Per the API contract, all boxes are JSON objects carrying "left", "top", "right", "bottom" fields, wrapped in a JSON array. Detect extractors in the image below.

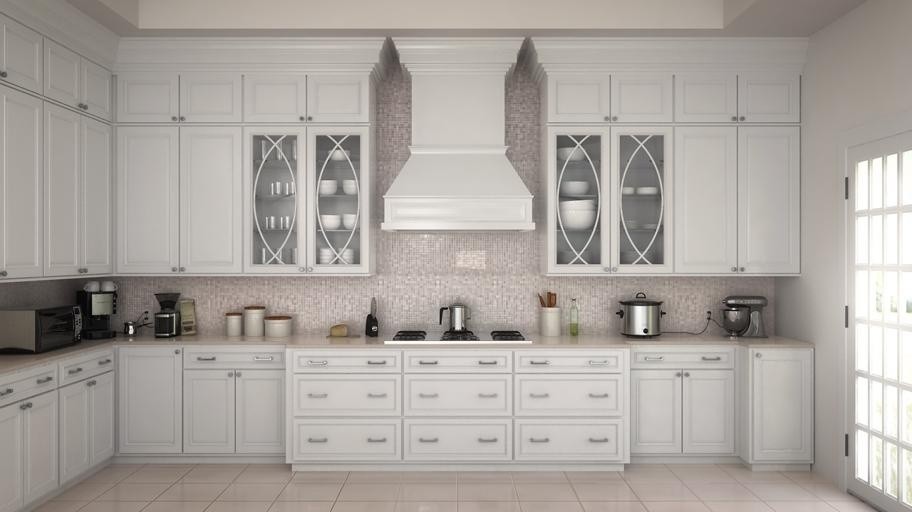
[{"left": 381, "top": 38, "right": 537, "bottom": 232}]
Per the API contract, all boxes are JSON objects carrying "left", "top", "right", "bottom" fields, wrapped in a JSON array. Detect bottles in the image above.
[
  {"left": 569, "top": 297, "right": 580, "bottom": 337},
  {"left": 226, "top": 312, "right": 242, "bottom": 337},
  {"left": 244, "top": 306, "right": 265, "bottom": 336}
]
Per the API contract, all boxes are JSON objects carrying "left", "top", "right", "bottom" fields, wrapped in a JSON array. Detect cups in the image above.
[
  {"left": 84, "top": 279, "right": 99, "bottom": 291},
  {"left": 101, "top": 280, "right": 117, "bottom": 292},
  {"left": 540, "top": 307, "right": 562, "bottom": 337},
  {"left": 343, "top": 213, "right": 359, "bottom": 230}
]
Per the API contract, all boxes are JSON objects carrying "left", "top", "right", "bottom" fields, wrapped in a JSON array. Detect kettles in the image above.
[{"left": 124, "top": 320, "right": 137, "bottom": 337}]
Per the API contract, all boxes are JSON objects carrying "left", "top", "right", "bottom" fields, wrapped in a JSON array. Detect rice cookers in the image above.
[{"left": 616, "top": 291, "right": 666, "bottom": 339}]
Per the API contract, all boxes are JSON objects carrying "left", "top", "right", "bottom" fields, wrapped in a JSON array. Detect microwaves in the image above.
[{"left": 0, "top": 304, "right": 83, "bottom": 355}]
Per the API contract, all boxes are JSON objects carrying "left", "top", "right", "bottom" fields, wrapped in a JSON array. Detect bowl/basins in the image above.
[
  {"left": 319, "top": 178, "right": 337, "bottom": 196},
  {"left": 320, "top": 248, "right": 335, "bottom": 264},
  {"left": 321, "top": 213, "right": 340, "bottom": 229},
  {"left": 623, "top": 220, "right": 638, "bottom": 230},
  {"left": 559, "top": 146, "right": 585, "bottom": 162},
  {"left": 339, "top": 248, "right": 354, "bottom": 265},
  {"left": 620, "top": 187, "right": 658, "bottom": 196},
  {"left": 560, "top": 251, "right": 592, "bottom": 265},
  {"left": 343, "top": 178, "right": 358, "bottom": 195},
  {"left": 328, "top": 148, "right": 350, "bottom": 161},
  {"left": 559, "top": 199, "right": 594, "bottom": 230},
  {"left": 560, "top": 180, "right": 590, "bottom": 197},
  {"left": 620, "top": 249, "right": 657, "bottom": 264}
]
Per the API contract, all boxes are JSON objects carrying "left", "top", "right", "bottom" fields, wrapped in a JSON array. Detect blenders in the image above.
[{"left": 719, "top": 295, "right": 769, "bottom": 341}]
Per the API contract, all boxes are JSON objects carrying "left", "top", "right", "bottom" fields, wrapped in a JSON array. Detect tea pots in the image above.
[{"left": 439, "top": 302, "right": 473, "bottom": 332}]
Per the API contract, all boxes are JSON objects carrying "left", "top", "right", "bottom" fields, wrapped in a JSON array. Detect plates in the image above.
[{"left": 644, "top": 223, "right": 660, "bottom": 229}]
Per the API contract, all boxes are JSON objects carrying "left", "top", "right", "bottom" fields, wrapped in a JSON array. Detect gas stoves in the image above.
[{"left": 392, "top": 330, "right": 524, "bottom": 340}]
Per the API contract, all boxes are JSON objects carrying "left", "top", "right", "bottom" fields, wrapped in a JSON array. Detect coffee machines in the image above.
[
  {"left": 76, "top": 291, "right": 117, "bottom": 339},
  {"left": 151, "top": 291, "right": 181, "bottom": 337}
]
